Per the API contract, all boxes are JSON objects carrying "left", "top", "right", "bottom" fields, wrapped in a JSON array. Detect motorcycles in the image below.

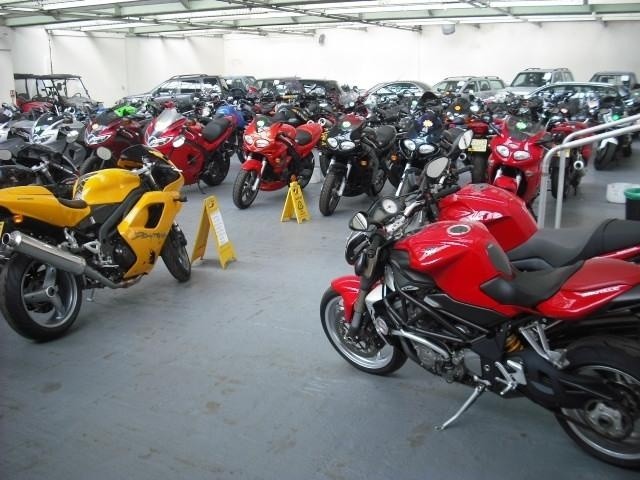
[
  {"left": 384, "top": 122, "right": 640, "bottom": 270},
  {"left": 0, "top": 131, "right": 194, "bottom": 344},
  {"left": 312, "top": 179, "right": 640, "bottom": 472}
]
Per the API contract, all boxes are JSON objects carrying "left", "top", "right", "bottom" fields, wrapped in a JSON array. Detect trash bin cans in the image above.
[{"left": 624, "top": 187, "right": 640, "bottom": 221}]
